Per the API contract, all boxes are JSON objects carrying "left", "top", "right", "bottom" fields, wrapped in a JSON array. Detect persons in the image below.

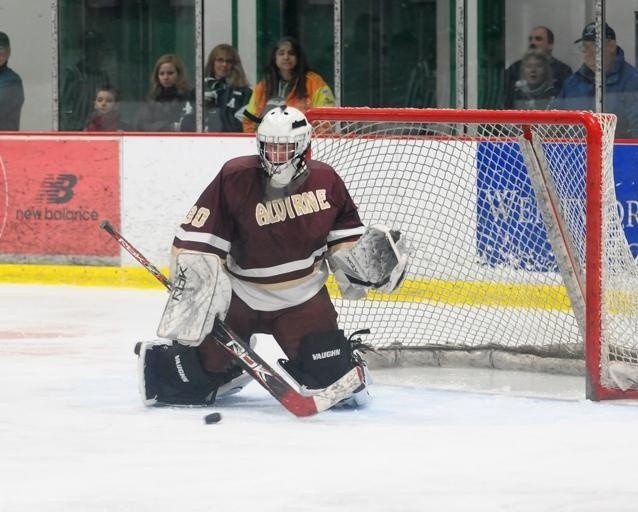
[
  {"left": 192, "top": 44, "right": 251, "bottom": 135},
  {"left": 507, "top": 50, "right": 557, "bottom": 111},
  {"left": 135, "top": 108, "right": 408, "bottom": 410},
  {"left": 0, "top": 29, "right": 25, "bottom": 131},
  {"left": 78, "top": 85, "right": 123, "bottom": 130},
  {"left": 557, "top": 22, "right": 638, "bottom": 98},
  {"left": 235, "top": 38, "right": 337, "bottom": 134},
  {"left": 150, "top": 55, "right": 197, "bottom": 102},
  {"left": 505, "top": 27, "right": 572, "bottom": 95}
]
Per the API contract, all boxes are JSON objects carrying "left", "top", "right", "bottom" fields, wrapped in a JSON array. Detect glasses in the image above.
[{"left": 215, "top": 56, "right": 232, "bottom": 65}]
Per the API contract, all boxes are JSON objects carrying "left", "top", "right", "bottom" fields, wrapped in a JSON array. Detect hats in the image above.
[{"left": 574, "top": 22, "right": 615, "bottom": 44}]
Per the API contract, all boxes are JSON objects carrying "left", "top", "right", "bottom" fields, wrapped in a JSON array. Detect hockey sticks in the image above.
[{"left": 100, "top": 220, "right": 364, "bottom": 418}]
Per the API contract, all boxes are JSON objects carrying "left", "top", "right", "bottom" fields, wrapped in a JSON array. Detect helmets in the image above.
[{"left": 257, "top": 108, "right": 311, "bottom": 182}]
